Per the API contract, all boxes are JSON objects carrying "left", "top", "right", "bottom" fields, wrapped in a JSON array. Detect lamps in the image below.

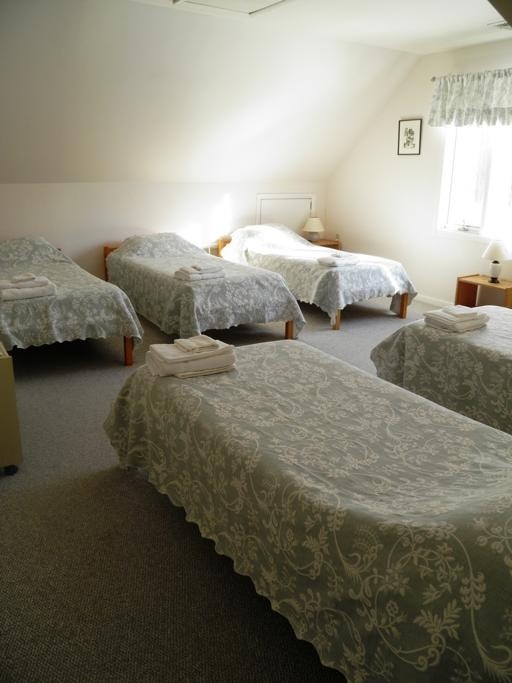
[
  {"left": 302, "top": 217, "right": 325, "bottom": 241},
  {"left": 480, "top": 238, "right": 509, "bottom": 283}
]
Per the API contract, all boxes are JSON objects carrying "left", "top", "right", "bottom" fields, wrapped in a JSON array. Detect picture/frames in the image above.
[{"left": 398, "top": 116, "right": 421, "bottom": 155}]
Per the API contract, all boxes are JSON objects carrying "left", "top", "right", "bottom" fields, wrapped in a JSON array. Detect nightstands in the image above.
[{"left": 455, "top": 273, "right": 510, "bottom": 308}]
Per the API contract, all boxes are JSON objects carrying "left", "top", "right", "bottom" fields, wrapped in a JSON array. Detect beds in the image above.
[
  {"left": 1, "top": 233, "right": 142, "bottom": 368},
  {"left": 368, "top": 302, "right": 512, "bottom": 434},
  {"left": 217, "top": 223, "right": 418, "bottom": 331},
  {"left": 102, "top": 232, "right": 307, "bottom": 342},
  {"left": 102, "top": 339, "right": 509, "bottom": 682}
]
